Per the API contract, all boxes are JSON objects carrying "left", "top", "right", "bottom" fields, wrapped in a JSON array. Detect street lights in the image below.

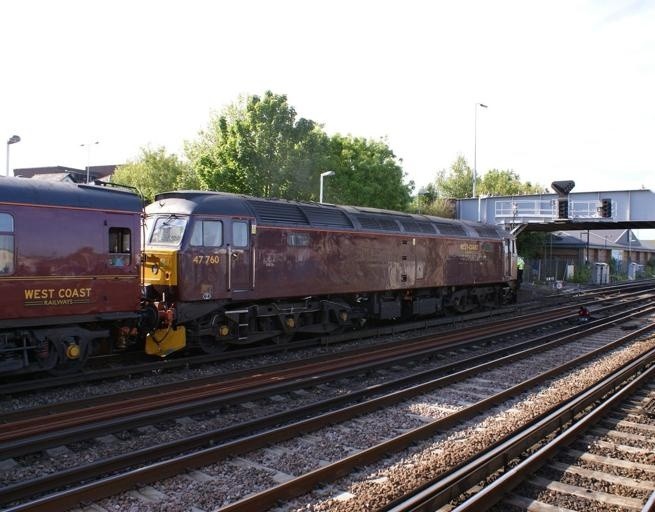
[
  {"left": 81, "top": 139, "right": 100, "bottom": 183},
  {"left": 6, "top": 135, "right": 22, "bottom": 177},
  {"left": 317, "top": 171, "right": 336, "bottom": 204},
  {"left": 473, "top": 100, "right": 490, "bottom": 222}
]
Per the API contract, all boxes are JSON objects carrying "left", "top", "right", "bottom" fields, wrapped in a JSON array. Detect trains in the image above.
[{"left": 0, "top": 177, "right": 519, "bottom": 375}]
